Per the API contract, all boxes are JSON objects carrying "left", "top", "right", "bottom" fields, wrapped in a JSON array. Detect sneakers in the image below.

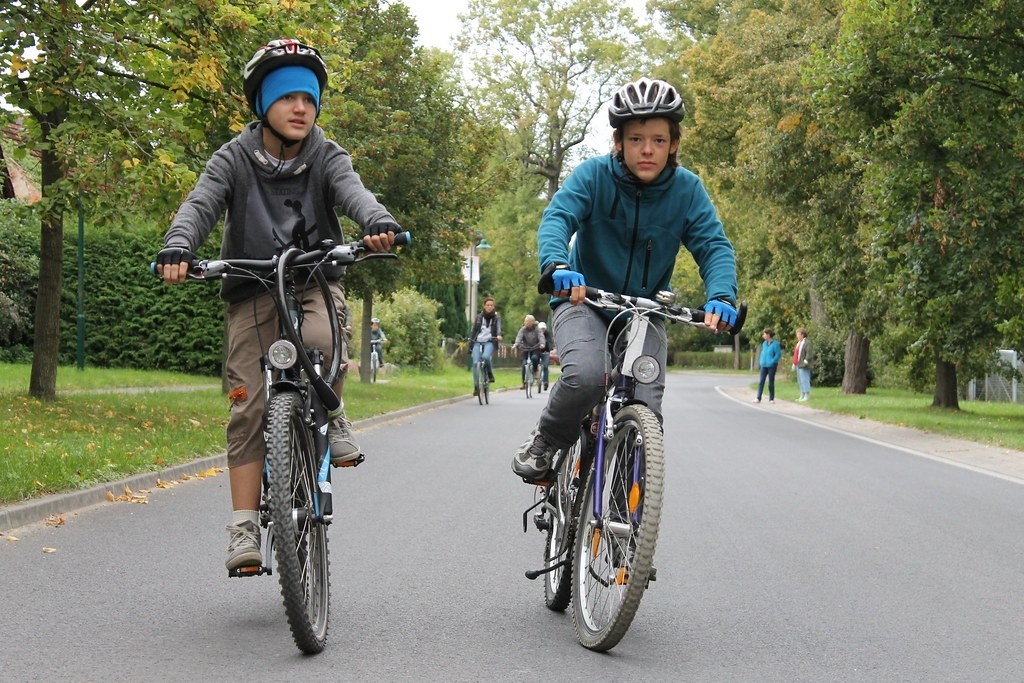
[
  {"left": 511, "top": 417, "right": 559, "bottom": 480},
  {"left": 606, "top": 534, "right": 658, "bottom": 578},
  {"left": 327, "top": 408, "right": 361, "bottom": 462},
  {"left": 225, "top": 519, "right": 263, "bottom": 571}
]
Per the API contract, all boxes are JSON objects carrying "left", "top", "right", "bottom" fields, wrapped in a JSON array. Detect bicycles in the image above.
[
  {"left": 522, "top": 261, "right": 747, "bottom": 651},
  {"left": 517, "top": 342, "right": 554, "bottom": 398},
  {"left": 151, "top": 231, "right": 411, "bottom": 655},
  {"left": 463, "top": 338, "right": 498, "bottom": 405},
  {"left": 371, "top": 339, "right": 386, "bottom": 382}
]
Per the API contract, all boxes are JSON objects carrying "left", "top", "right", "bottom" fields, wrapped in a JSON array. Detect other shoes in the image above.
[
  {"left": 535, "top": 371, "right": 540, "bottom": 379},
  {"left": 490, "top": 377, "right": 494, "bottom": 382},
  {"left": 544, "top": 383, "right": 548, "bottom": 390},
  {"left": 520, "top": 383, "right": 526, "bottom": 390},
  {"left": 474, "top": 389, "right": 480, "bottom": 396},
  {"left": 796, "top": 394, "right": 810, "bottom": 402}
]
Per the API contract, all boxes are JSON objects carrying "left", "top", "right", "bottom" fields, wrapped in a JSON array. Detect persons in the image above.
[
  {"left": 512, "top": 314, "right": 545, "bottom": 390},
  {"left": 469, "top": 298, "right": 502, "bottom": 396},
  {"left": 534, "top": 322, "right": 553, "bottom": 390},
  {"left": 511, "top": 78, "right": 739, "bottom": 581},
  {"left": 368, "top": 318, "right": 389, "bottom": 370},
  {"left": 753, "top": 330, "right": 781, "bottom": 404},
  {"left": 156, "top": 38, "right": 402, "bottom": 571},
  {"left": 792, "top": 328, "right": 812, "bottom": 402}
]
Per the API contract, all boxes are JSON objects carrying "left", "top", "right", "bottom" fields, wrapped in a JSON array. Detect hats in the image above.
[
  {"left": 255, "top": 66, "right": 320, "bottom": 119},
  {"left": 538, "top": 322, "right": 546, "bottom": 329}
]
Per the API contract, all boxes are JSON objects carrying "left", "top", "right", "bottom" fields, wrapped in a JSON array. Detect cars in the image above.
[{"left": 549, "top": 349, "right": 559, "bottom": 365}]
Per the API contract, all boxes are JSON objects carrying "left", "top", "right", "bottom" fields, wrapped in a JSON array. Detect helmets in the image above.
[
  {"left": 371, "top": 318, "right": 380, "bottom": 324},
  {"left": 609, "top": 77, "right": 685, "bottom": 128},
  {"left": 243, "top": 39, "right": 327, "bottom": 115}
]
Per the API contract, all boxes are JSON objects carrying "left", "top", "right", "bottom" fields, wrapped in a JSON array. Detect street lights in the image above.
[{"left": 467, "top": 231, "right": 492, "bottom": 371}]
